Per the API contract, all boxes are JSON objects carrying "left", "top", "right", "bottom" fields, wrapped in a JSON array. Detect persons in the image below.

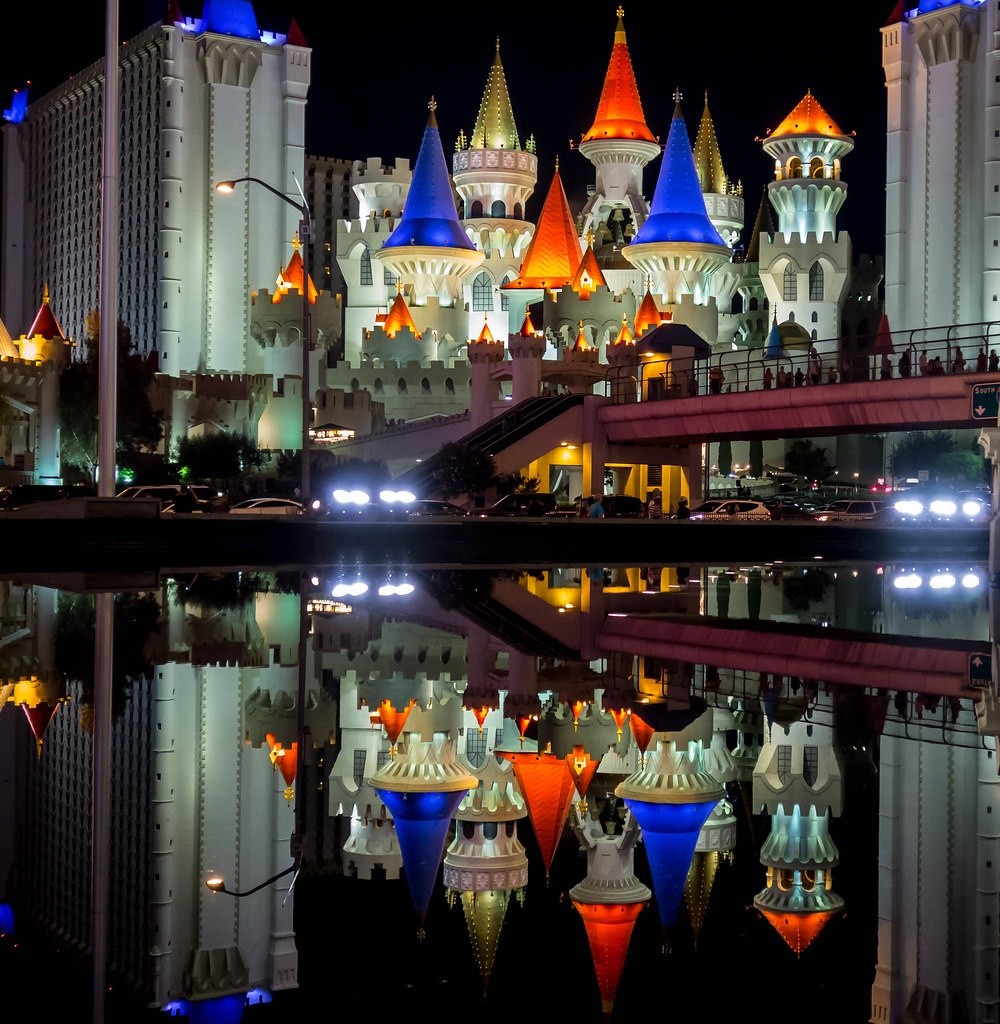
[
  {"left": 543, "top": 388, "right": 573, "bottom": 397},
  {"left": 878, "top": 347, "right": 1000, "bottom": 378},
  {"left": 649, "top": 493, "right": 664, "bottom": 519},
  {"left": 684, "top": 360, "right": 839, "bottom": 395},
  {"left": 677, "top": 496, "right": 690, "bottom": 520},
  {"left": 382, "top": 613, "right": 466, "bottom": 642},
  {"left": 536, "top": 656, "right": 569, "bottom": 668},
  {"left": 171, "top": 484, "right": 302, "bottom": 513},
  {"left": 384, "top": 408, "right": 470, "bottom": 431},
  {"left": 657, "top": 658, "right": 977, "bottom": 725},
  {"left": 166, "top": 573, "right": 302, "bottom": 599},
  {"left": 588, "top": 492, "right": 606, "bottom": 521}
]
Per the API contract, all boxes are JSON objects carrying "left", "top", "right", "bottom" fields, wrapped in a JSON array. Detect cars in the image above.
[
  {"left": 115, "top": 484, "right": 211, "bottom": 504},
  {"left": 566, "top": 494, "right": 643, "bottom": 516},
  {"left": 815, "top": 500, "right": 885, "bottom": 522},
  {"left": 161, "top": 499, "right": 232, "bottom": 513},
  {"left": 384, "top": 500, "right": 468, "bottom": 517},
  {"left": 467, "top": 493, "right": 559, "bottom": 518},
  {"left": 0, "top": 484, "right": 95, "bottom": 509},
  {"left": 958, "top": 487, "right": 991, "bottom": 495},
  {"left": 803, "top": 503, "right": 837, "bottom": 516},
  {"left": 671, "top": 499, "right": 771, "bottom": 520},
  {"left": 229, "top": 498, "right": 302, "bottom": 514}
]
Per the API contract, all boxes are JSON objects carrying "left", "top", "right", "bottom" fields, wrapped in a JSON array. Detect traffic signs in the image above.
[
  {"left": 969, "top": 652, "right": 993, "bottom": 691},
  {"left": 972, "top": 383, "right": 1000, "bottom": 419}
]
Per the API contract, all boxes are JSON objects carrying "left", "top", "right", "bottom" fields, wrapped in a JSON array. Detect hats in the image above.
[
  {"left": 594, "top": 494, "right": 603, "bottom": 500},
  {"left": 679, "top": 496, "right": 687, "bottom": 502},
  {"left": 653, "top": 488, "right": 662, "bottom": 497}
]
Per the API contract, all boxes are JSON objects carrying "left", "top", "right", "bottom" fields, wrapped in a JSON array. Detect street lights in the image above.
[
  {"left": 854, "top": 473, "right": 859, "bottom": 493},
  {"left": 215, "top": 176, "right": 311, "bottom": 508},
  {"left": 835, "top": 471, "right": 838, "bottom": 495},
  {"left": 205, "top": 572, "right": 309, "bottom": 897}
]
